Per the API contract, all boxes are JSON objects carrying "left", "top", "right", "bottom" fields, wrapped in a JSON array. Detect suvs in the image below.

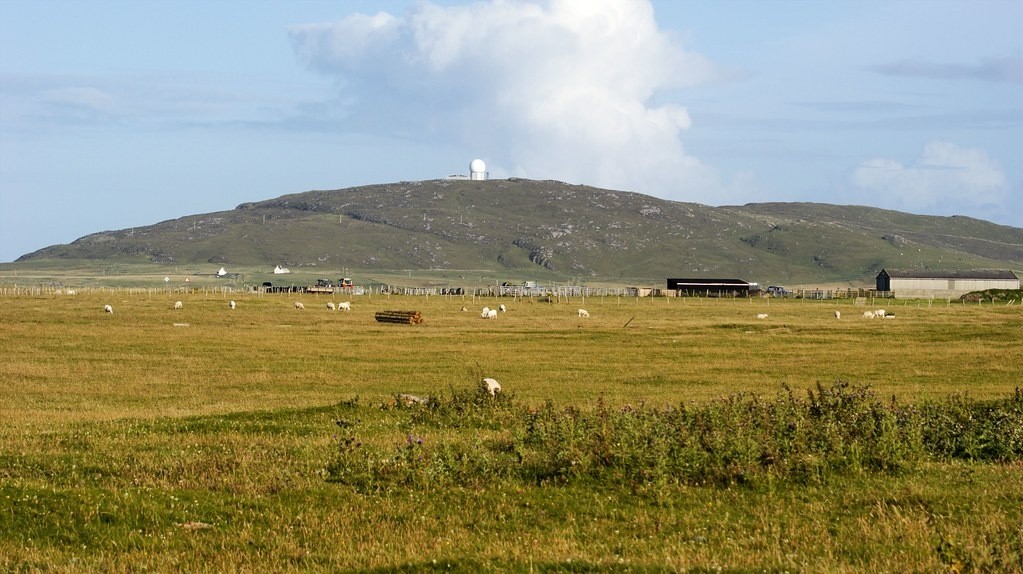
[{"left": 767, "top": 285, "right": 793, "bottom": 298}]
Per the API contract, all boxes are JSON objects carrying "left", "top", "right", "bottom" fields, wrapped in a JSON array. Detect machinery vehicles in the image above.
[
  {"left": 308, "top": 278, "right": 333, "bottom": 293},
  {"left": 339, "top": 277, "right": 352, "bottom": 288}
]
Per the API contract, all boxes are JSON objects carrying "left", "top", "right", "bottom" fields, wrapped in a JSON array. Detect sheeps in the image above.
[
  {"left": 756, "top": 314, "right": 768, "bottom": 319},
  {"left": 174, "top": 300, "right": 182, "bottom": 309},
  {"left": 834, "top": 311, "right": 840, "bottom": 319},
  {"left": 105, "top": 305, "right": 113, "bottom": 314},
  {"left": 499, "top": 304, "right": 506, "bottom": 313},
  {"left": 488, "top": 310, "right": 497, "bottom": 319},
  {"left": 326, "top": 302, "right": 335, "bottom": 310},
  {"left": 861, "top": 311, "right": 873, "bottom": 319},
  {"left": 229, "top": 301, "right": 236, "bottom": 309},
  {"left": 578, "top": 309, "right": 589, "bottom": 318},
  {"left": 338, "top": 303, "right": 350, "bottom": 311},
  {"left": 295, "top": 302, "right": 304, "bottom": 310},
  {"left": 480, "top": 307, "right": 489, "bottom": 317},
  {"left": 872, "top": 310, "right": 885, "bottom": 318}
]
